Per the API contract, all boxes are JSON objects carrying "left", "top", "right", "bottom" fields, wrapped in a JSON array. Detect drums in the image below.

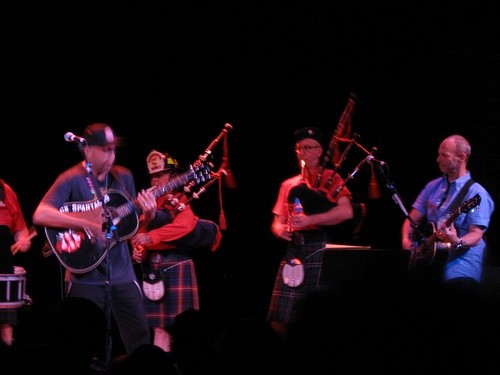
[{"left": 1, "top": 262, "right": 28, "bottom": 310}]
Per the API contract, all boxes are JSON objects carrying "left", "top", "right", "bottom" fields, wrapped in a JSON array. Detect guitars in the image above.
[
  {"left": 409, "top": 193, "right": 482, "bottom": 274},
  {"left": 44, "top": 163, "right": 213, "bottom": 276}
]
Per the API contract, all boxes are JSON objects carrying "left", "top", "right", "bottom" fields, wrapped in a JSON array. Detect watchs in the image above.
[{"left": 456, "top": 239, "right": 464, "bottom": 249}]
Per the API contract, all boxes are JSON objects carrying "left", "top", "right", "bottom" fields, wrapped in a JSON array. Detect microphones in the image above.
[
  {"left": 63, "top": 132, "right": 86, "bottom": 143},
  {"left": 365, "top": 156, "right": 385, "bottom": 166}
]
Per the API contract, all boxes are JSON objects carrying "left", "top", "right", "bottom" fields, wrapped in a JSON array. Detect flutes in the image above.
[
  {"left": 145, "top": 122, "right": 236, "bottom": 232},
  {"left": 285, "top": 93, "right": 385, "bottom": 246}
]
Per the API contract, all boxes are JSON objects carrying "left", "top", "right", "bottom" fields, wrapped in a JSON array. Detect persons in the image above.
[
  {"left": 33, "top": 124, "right": 158, "bottom": 371},
  {"left": 130, "top": 149, "right": 199, "bottom": 352},
  {"left": 402, "top": 135, "right": 494, "bottom": 283},
  {"left": 266, "top": 129, "right": 353, "bottom": 333},
  {"left": 0, "top": 180, "right": 30, "bottom": 345}
]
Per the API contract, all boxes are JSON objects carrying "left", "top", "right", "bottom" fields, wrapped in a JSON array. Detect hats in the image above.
[
  {"left": 82, "top": 124, "right": 124, "bottom": 146},
  {"left": 294, "top": 126, "right": 326, "bottom": 150},
  {"left": 145, "top": 149, "right": 179, "bottom": 176}
]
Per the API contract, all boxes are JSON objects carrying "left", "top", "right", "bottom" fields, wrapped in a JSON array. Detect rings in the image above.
[{"left": 144, "top": 202, "right": 149, "bottom": 207}]
